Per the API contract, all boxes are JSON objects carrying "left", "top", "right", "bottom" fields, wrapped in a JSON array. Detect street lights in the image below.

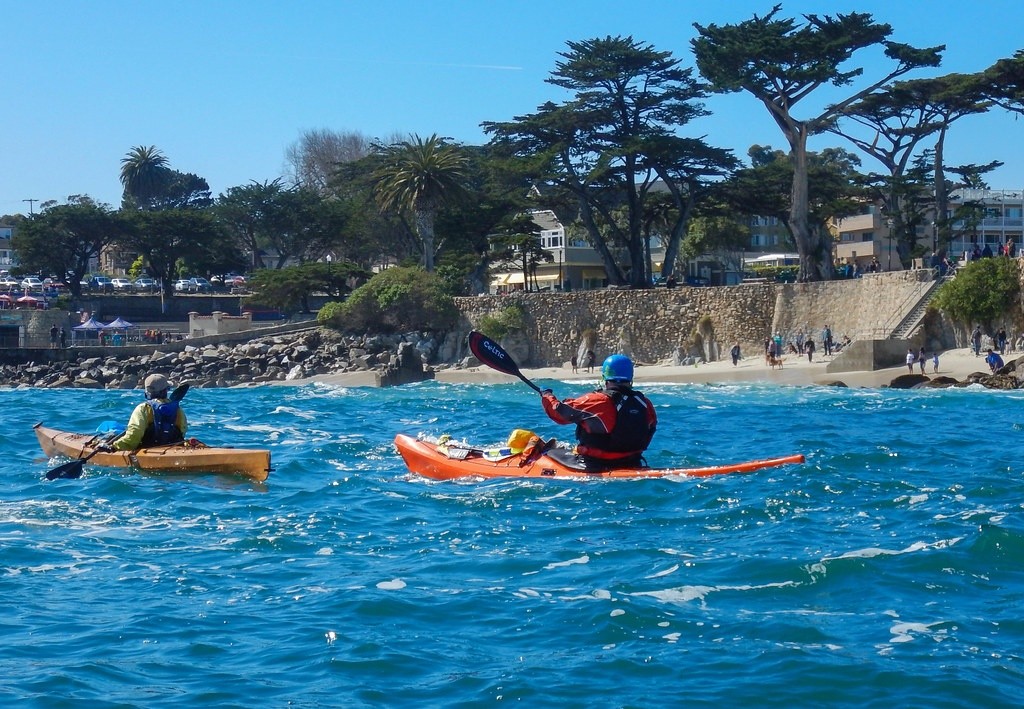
[
  {"left": 931, "top": 222, "right": 936, "bottom": 253},
  {"left": 326, "top": 254, "right": 332, "bottom": 281},
  {"left": 247, "top": 251, "right": 254, "bottom": 264},
  {"left": 559, "top": 247, "right": 562, "bottom": 290},
  {"left": 888, "top": 219, "right": 893, "bottom": 272},
  {"left": 103, "top": 269, "right": 108, "bottom": 295}
]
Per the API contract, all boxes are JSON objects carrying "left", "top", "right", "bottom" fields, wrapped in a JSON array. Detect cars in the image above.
[{"left": 0, "top": 269, "right": 251, "bottom": 293}]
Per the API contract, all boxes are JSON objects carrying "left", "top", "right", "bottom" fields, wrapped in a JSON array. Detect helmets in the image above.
[{"left": 601, "top": 354, "right": 634, "bottom": 381}]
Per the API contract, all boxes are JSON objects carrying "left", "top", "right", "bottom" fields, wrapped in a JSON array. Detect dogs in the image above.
[{"left": 767, "top": 353, "right": 787, "bottom": 370}]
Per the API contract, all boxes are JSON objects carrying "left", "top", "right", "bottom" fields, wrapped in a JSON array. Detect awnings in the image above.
[
  {"left": 490, "top": 269, "right": 559, "bottom": 286},
  {"left": 583, "top": 269, "right": 606, "bottom": 278}
]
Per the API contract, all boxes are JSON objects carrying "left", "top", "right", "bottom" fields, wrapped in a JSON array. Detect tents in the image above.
[{"left": 72, "top": 314, "right": 133, "bottom": 339}]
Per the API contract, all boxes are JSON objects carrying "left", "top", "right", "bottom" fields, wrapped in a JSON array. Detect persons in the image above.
[
  {"left": 787, "top": 342, "right": 797, "bottom": 354},
  {"left": 730, "top": 342, "right": 740, "bottom": 365},
  {"left": 571, "top": 355, "right": 578, "bottom": 374},
  {"left": 50, "top": 324, "right": 66, "bottom": 349},
  {"left": 822, "top": 324, "right": 850, "bottom": 355},
  {"left": 845, "top": 256, "right": 881, "bottom": 279},
  {"left": 667, "top": 275, "right": 677, "bottom": 288},
  {"left": 930, "top": 238, "right": 1016, "bottom": 274},
  {"left": 440, "top": 355, "right": 657, "bottom": 471},
  {"left": 95, "top": 374, "right": 188, "bottom": 452},
  {"left": 804, "top": 337, "right": 815, "bottom": 362},
  {"left": 764, "top": 331, "right": 782, "bottom": 358},
  {"left": 970, "top": 325, "right": 1012, "bottom": 372},
  {"left": 99, "top": 329, "right": 121, "bottom": 346},
  {"left": 796, "top": 328, "right": 804, "bottom": 354},
  {"left": 587, "top": 350, "right": 596, "bottom": 373},
  {"left": 906, "top": 347, "right": 938, "bottom": 375},
  {"left": 145, "top": 328, "right": 182, "bottom": 344}
]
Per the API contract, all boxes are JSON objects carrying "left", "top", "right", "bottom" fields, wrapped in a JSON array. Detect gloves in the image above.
[
  {"left": 95, "top": 443, "right": 112, "bottom": 453},
  {"left": 537, "top": 386, "right": 553, "bottom": 396}
]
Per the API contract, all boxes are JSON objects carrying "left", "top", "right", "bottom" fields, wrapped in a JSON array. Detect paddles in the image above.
[
  {"left": 44, "top": 382, "right": 191, "bottom": 480},
  {"left": 467, "top": 329, "right": 544, "bottom": 394}
]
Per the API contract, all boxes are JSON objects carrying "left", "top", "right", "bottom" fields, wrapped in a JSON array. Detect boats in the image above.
[
  {"left": 34, "top": 422, "right": 271, "bottom": 483},
  {"left": 395, "top": 433, "right": 805, "bottom": 479}
]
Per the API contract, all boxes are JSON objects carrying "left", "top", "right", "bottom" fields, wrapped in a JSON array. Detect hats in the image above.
[{"left": 145, "top": 374, "right": 174, "bottom": 392}]
